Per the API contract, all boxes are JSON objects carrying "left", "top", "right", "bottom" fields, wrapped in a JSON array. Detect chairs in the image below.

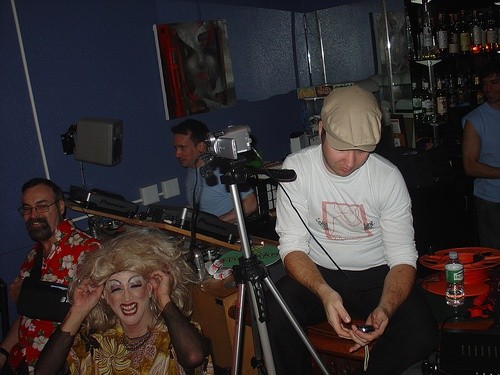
[{"left": 421, "top": 329, "right": 500, "bottom": 375}]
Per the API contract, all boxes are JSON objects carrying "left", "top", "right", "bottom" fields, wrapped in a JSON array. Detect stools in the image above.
[{"left": 301, "top": 315, "right": 379, "bottom": 375}]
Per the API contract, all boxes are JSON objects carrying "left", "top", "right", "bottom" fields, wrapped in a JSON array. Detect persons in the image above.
[
  {"left": 462, "top": 62, "right": 500, "bottom": 250},
  {"left": 171, "top": 119, "right": 256, "bottom": 224},
  {"left": 259, "top": 85, "right": 438, "bottom": 375},
  {"left": 0, "top": 177, "right": 215, "bottom": 375}
]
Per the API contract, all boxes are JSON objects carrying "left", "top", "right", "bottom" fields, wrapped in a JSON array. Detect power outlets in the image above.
[
  {"left": 140, "top": 185, "right": 161, "bottom": 206},
  {"left": 161, "top": 177, "right": 181, "bottom": 199}
]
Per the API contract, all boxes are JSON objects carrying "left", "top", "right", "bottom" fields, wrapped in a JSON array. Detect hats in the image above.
[{"left": 321, "top": 86, "right": 382, "bottom": 152}]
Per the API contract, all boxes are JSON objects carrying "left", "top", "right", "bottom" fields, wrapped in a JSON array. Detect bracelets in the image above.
[{"left": 0, "top": 347, "right": 9, "bottom": 357}]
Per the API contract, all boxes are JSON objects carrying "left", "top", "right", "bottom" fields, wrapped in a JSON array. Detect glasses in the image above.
[{"left": 17, "top": 202, "right": 56, "bottom": 215}]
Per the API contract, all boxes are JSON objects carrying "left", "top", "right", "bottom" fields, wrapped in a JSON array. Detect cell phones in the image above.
[{"left": 347, "top": 324, "right": 375, "bottom": 333}]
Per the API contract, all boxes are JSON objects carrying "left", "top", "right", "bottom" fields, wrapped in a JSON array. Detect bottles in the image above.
[
  {"left": 402, "top": 2, "right": 500, "bottom": 61},
  {"left": 444, "top": 251, "right": 465, "bottom": 308},
  {"left": 391, "top": 66, "right": 487, "bottom": 151}
]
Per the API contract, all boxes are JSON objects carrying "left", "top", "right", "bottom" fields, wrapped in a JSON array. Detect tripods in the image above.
[{"left": 217, "top": 162, "right": 330, "bottom": 375}]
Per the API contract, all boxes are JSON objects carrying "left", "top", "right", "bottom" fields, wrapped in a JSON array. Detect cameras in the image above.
[{"left": 204, "top": 123, "right": 252, "bottom": 160}]
